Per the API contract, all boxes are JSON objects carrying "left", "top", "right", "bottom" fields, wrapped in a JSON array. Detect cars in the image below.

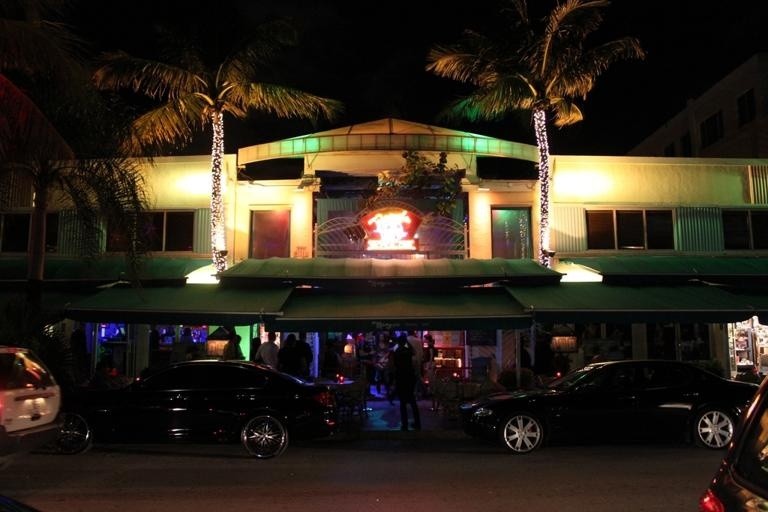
[
  {"left": 22, "top": 358, "right": 343, "bottom": 462},
  {"left": 459, "top": 359, "right": 761, "bottom": 452}
]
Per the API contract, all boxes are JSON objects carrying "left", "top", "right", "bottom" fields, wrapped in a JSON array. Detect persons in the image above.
[
  {"left": 223, "top": 335, "right": 244, "bottom": 362},
  {"left": 297, "top": 333, "right": 313, "bottom": 366},
  {"left": 320, "top": 330, "right": 437, "bottom": 433},
  {"left": 278, "top": 333, "right": 307, "bottom": 380},
  {"left": 256, "top": 331, "right": 279, "bottom": 369},
  {"left": 180, "top": 328, "right": 194, "bottom": 360}
]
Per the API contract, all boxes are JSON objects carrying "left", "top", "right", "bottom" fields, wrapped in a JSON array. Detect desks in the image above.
[
  {"left": 317, "top": 382, "right": 368, "bottom": 427},
  {"left": 432, "top": 382, "right": 481, "bottom": 416}
]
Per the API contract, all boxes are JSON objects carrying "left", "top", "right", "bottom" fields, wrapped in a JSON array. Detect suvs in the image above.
[
  {"left": 0, "top": 345, "right": 61, "bottom": 460},
  {"left": 697, "top": 372, "right": 768, "bottom": 512}
]
